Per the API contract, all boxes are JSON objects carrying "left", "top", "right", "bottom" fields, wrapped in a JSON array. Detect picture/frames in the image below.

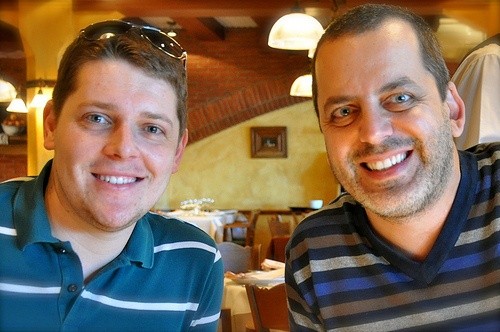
[{"left": 250, "top": 126, "right": 286, "bottom": 158}]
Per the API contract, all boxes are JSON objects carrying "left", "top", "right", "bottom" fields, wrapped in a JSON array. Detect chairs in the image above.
[{"left": 215, "top": 207, "right": 319, "bottom": 332}]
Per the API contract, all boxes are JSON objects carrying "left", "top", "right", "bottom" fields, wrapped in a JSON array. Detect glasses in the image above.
[{"left": 59, "top": 21, "right": 188, "bottom": 79}]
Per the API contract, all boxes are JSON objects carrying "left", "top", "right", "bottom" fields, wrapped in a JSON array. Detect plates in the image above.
[{"left": 233, "top": 277, "right": 285, "bottom": 284}]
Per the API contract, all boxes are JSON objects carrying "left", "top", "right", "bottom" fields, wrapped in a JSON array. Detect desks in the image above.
[
  {"left": 162, "top": 210, "right": 248, "bottom": 242},
  {"left": 221, "top": 271, "right": 285, "bottom": 332}
]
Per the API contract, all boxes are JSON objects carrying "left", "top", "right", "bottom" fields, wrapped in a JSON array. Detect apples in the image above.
[{"left": 3, "top": 113, "right": 24, "bottom": 126}]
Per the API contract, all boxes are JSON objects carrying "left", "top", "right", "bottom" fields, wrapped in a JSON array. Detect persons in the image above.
[
  {"left": 0, "top": 20, "right": 224, "bottom": 332},
  {"left": 285, "top": 3, "right": 500, "bottom": 332},
  {"left": 449, "top": 34, "right": 500, "bottom": 151}
]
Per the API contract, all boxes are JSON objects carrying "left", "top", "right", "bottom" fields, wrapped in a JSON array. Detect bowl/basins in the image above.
[
  {"left": 1, "top": 123, "right": 25, "bottom": 136},
  {"left": 309, "top": 200, "right": 323, "bottom": 210}
]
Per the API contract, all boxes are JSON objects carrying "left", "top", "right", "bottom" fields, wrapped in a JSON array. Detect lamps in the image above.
[
  {"left": 267, "top": 0, "right": 324, "bottom": 50},
  {"left": 0, "top": 74, "right": 48, "bottom": 113},
  {"left": 289, "top": 59, "right": 312, "bottom": 97}
]
